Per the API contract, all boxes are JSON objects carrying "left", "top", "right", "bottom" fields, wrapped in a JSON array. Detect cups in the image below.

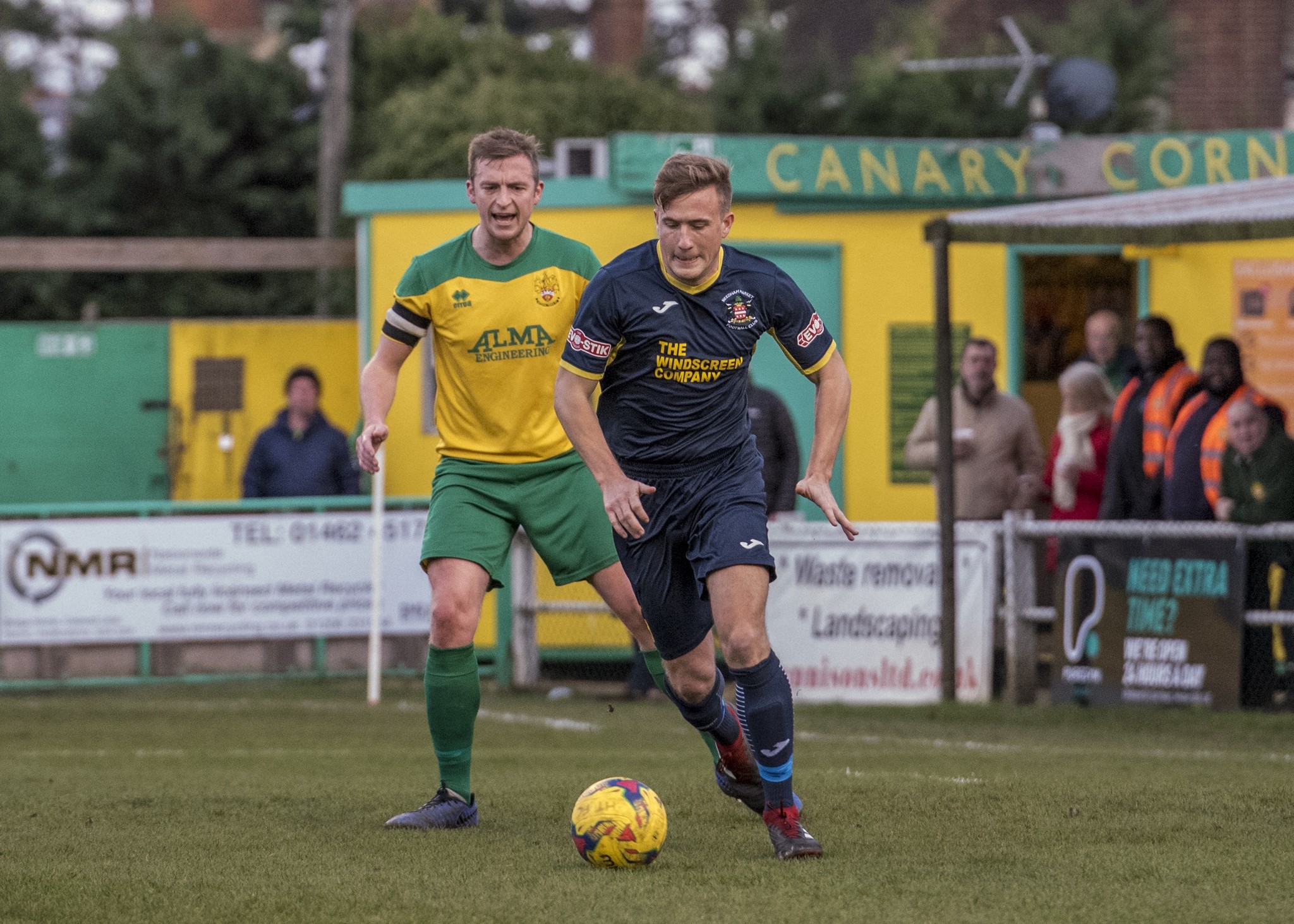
[{"left": 952, "top": 428, "right": 973, "bottom": 441}]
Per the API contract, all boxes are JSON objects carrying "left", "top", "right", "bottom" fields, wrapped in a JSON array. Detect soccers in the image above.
[{"left": 569, "top": 776, "right": 667, "bottom": 868}]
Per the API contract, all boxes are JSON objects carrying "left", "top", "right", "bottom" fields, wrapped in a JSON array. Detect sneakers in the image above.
[
  {"left": 767, "top": 814, "right": 824, "bottom": 860},
  {"left": 384, "top": 788, "right": 478, "bottom": 829},
  {"left": 714, "top": 699, "right": 760, "bottom": 786},
  {"left": 714, "top": 765, "right": 804, "bottom": 819}
]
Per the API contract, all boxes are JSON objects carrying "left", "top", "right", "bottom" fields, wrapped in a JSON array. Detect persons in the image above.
[
  {"left": 354, "top": 129, "right": 806, "bottom": 834},
  {"left": 1102, "top": 313, "right": 1202, "bottom": 522},
  {"left": 1212, "top": 398, "right": 1294, "bottom": 708},
  {"left": 1081, "top": 307, "right": 1139, "bottom": 394},
  {"left": 237, "top": 361, "right": 361, "bottom": 501},
  {"left": 1016, "top": 360, "right": 1114, "bottom": 572},
  {"left": 902, "top": 336, "right": 1044, "bottom": 703},
  {"left": 558, "top": 148, "right": 865, "bottom": 862},
  {"left": 1158, "top": 331, "right": 1286, "bottom": 520},
  {"left": 737, "top": 371, "right": 802, "bottom": 521}
]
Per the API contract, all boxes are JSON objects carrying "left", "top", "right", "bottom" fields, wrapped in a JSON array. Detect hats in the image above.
[{"left": 286, "top": 368, "right": 319, "bottom": 395}]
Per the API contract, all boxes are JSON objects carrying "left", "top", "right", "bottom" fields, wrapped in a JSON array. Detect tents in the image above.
[{"left": 918, "top": 169, "right": 1294, "bottom": 705}]
